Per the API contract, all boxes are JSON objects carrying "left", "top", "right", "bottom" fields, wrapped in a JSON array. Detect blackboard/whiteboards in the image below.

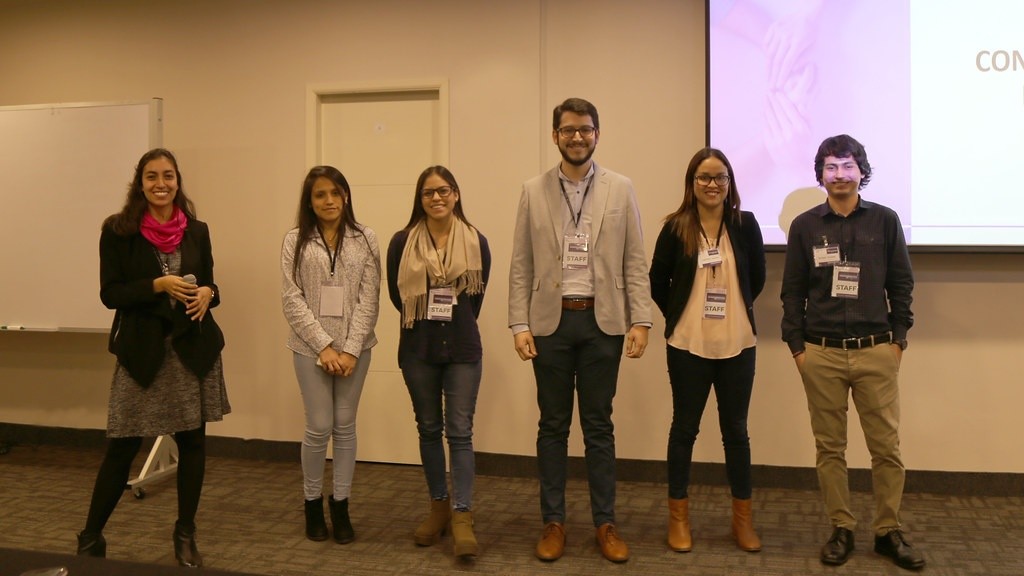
[{"left": 0, "top": 97, "right": 165, "bottom": 333}]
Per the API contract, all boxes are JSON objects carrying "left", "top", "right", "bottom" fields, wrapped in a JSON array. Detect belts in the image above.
[
  {"left": 803, "top": 333, "right": 894, "bottom": 351},
  {"left": 562, "top": 298, "right": 595, "bottom": 310}
]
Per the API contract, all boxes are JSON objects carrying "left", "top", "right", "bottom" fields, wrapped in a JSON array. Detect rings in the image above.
[{"left": 641, "top": 349, "right": 643, "bottom": 351}]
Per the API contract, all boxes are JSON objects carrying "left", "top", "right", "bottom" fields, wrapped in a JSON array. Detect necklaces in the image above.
[
  {"left": 329, "top": 233, "right": 336, "bottom": 247},
  {"left": 436, "top": 244, "right": 444, "bottom": 253},
  {"left": 710, "top": 237, "right": 716, "bottom": 246}
]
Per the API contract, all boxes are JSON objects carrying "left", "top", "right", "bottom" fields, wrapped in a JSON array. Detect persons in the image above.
[
  {"left": 386, "top": 165, "right": 491, "bottom": 555},
  {"left": 779, "top": 133, "right": 926, "bottom": 571},
  {"left": 508, "top": 97, "right": 654, "bottom": 565},
  {"left": 280, "top": 165, "right": 382, "bottom": 545},
  {"left": 649, "top": 147, "right": 767, "bottom": 552},
  {"left": 75, "top": 149, "right": 233, "bottom": 568}
]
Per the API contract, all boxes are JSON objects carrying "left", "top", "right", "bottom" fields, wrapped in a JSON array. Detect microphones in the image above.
[{"left": 183, "top": 274, "right": 201, "bottom": 335}]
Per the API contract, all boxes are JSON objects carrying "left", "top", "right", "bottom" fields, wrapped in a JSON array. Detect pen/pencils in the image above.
[{"left": 0, "top": 325, "right": 24, "bottom": 330}]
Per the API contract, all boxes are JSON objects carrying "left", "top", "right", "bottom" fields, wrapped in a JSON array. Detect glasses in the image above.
[
  {"left": 419, "top": 186, "right": 456, "bottom": 199},
  {"left": 693, "top": 173, "right": 731, "bottom": 187},
  {"left": 557, "top": 124, "right": 598, "bottom": 138}
]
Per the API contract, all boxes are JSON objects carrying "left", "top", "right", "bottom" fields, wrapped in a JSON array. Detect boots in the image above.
[
  {"left": 304, "top": 493, "right": 328, "bottom": 541},
  {"left": 413, "top": 494, "right": 451, "bottom": 546},
  {"left": 329, "top": 493, "right": 355, "bottom": 543},
  {"left": 731, "top": 496, "right": 762, "bottom": 552},
  {"left": 668, "top": 497, "right": 691, "bottom": 552},
  {"left": 450, "top": 511, "right": 480, "bottom": 557}
]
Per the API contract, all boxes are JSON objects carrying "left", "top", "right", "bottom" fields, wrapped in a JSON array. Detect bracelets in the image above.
[
  {"left": 793, "top": 352, "right": 803, "bottom": 358},
  {"left": 211, "top": 291, "right": 214, "bottom": 298}
]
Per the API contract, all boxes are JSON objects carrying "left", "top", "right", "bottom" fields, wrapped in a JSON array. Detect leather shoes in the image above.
[
  {"left": 77, "top": 529, "right": 107, "bottom": 557},
  {"left": 536, "top": 521, "right": 566, "bottom": 561},
  {"left": 820, "top": 526, "right": 855, "bottom": 565},
  {"left": 874, "top": 529, "right": 926, "bottom": 570},
  {"left": 596, "top": 522, "right": 629, "bottom": 562},
  {"left": 173, "top": 520, "right": 203, "bottom": 569}
]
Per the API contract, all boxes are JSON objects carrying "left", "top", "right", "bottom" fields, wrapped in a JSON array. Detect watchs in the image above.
[{"left": 894, "top": 339, "right": 908, "bottom": 350}]
